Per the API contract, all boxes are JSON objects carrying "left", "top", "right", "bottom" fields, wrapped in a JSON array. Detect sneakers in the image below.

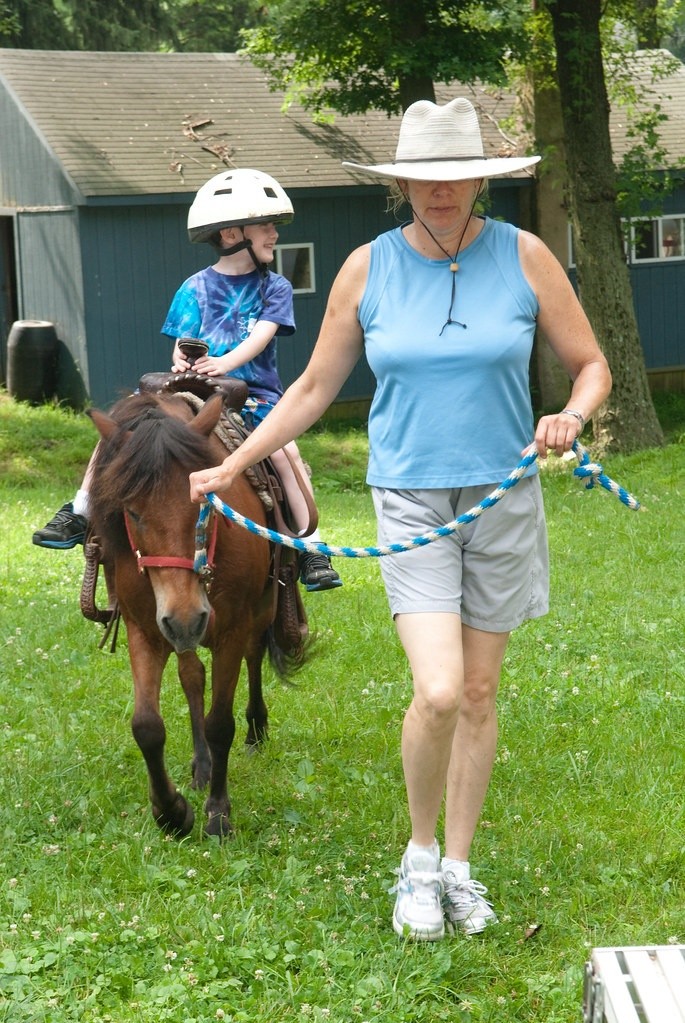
[
  {"left": 392, "top": 850, "right": 447, "bottom": 941},
  {"left": 300, "top": 541, "right": 342, "bottom": 591},
  {"left": 31, "top": 503, "right": 87, "bottom": 550},
  {"left": 440, "top": 872, "right": 499, "bottom": 935}
]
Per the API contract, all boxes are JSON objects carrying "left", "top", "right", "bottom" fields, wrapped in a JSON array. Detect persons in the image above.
[
  {"left": 31, "top": 166, "right": 347, "bottom": 592},
  {"left": 187, "top": 97, "right": 613, "bottom": 942}
]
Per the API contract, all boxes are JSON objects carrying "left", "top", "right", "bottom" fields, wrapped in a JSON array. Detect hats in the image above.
[{"left": 341, "top": 97, "right": 541, "bottom": 182}]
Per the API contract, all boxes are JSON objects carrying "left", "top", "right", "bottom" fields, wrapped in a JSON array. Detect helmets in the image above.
[{"left": 186, "top": 168, "right": 296, "bottom": 243}]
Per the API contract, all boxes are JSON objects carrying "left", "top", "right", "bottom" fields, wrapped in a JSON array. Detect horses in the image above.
[{"left": 83, "top": 383, "right": 311, "bottom": 839}]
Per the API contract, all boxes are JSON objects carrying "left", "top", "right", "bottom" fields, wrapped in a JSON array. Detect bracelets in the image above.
[{"left": 559, "top": 409, "right": 585, "bottom": 437}]
